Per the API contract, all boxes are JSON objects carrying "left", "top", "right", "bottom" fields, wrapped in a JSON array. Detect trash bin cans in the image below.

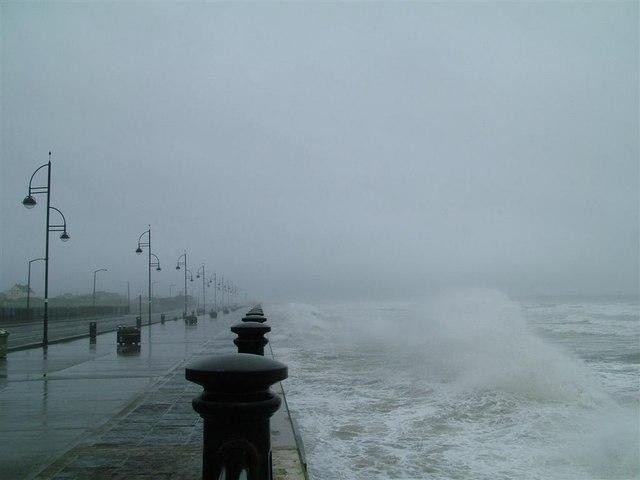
[
  {"left": 161, "top": 314, "right": 164, "bottom": 324},
  {"left": 136, "top": 317, "right": 141, "bottom": 327},
  {"left": 0, "top": 329, "right": 10, "bottom": 357},
  {"left": 90, "top": 321, "right": 97, "bottom": 338}
]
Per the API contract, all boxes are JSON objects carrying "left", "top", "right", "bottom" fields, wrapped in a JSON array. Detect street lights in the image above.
[
  {"left": 210, "top": 271, "right": 241, "bottom": 313},
  {"left": 197, "top": 261, "right": 212, "bottom": 316},
  {"left": 136, "top": 223, "right": 163, "bottom": 328},
  {"left": 175, "top": 251, "right": 195, "bottom": 320},
  {"left": 169, "top": 283, "right": 176, "bottom": 298},
  {"left": 151, "top": 280, "right": 160, "bottom": 311},
  {"left": 23, "top": 152, "right": 72, "bottom": 351},
  {"left": 93, "top": 268, "right": 108, "bottom": 318},
  {"left": 26, "top": 256, "right": 49, "bottom": 320}
]
